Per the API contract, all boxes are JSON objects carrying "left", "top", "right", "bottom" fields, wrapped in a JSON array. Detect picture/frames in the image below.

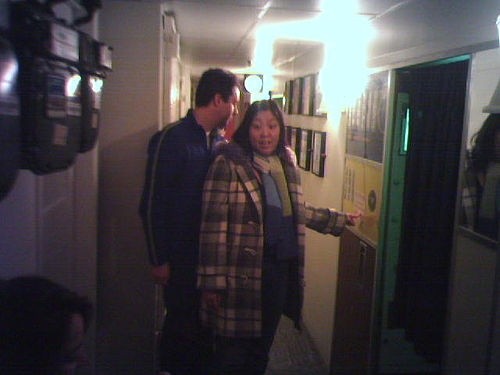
[{"left": 281, "top": 74, "right": 326, "bottom": 178}]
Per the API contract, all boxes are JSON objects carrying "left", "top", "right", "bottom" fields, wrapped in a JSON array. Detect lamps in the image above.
[{"left": 242, "top": 74, "right": 265, "bottom": 94}]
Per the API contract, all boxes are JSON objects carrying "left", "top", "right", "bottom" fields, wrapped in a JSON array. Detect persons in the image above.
[
  {"left": 195, "top": 100, "right": 362, "bottom": 375},
  {"left": 140, "top": 68, "right": 237, "bottom": 367},
  {"left": 0, "top": 277, "right": 94, "bottom": 375},
  {"left": 461, "top": 113, "right": 500, "bottom": 238}
]
